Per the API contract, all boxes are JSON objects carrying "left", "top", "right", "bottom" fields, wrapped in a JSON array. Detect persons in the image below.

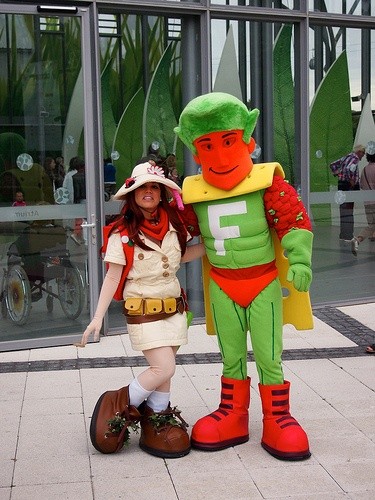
[
  {"left": 174, "top": 91, "right": 314, "bottom": 462},
  {"left": 350, "top": 151, "right": 375, "bottom": 256},
  {"left": 330, "top": 145, "right": 365, "bottom": 242},
  {"left": 7, "top": 201, "right": 66, "bottom": 289},
  {"left": 82, "top": 160, "right": 205, "bottom": 458},
  {"left": 46, "top": 156, "right": 66, "bottom": 189},
  {"left": 136, "top": 142, "right": 178, "bottom": 184},
  {"left": 12, "top": 192, "right": 25, "bottom": 206},
  {"left": 63, "top": 157, "right": 87, "bottom": 246},
  {"left": 104, "top": 158, "right": 115, "bottom": 183}
]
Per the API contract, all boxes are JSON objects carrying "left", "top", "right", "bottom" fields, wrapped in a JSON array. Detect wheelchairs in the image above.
[{"left": 0, "top": 225, "right": 85, "bottom": 326}]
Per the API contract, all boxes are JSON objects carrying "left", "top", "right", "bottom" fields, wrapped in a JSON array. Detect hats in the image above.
[{"left": 113, "top": 161, "right": 182, "bottom": 199}]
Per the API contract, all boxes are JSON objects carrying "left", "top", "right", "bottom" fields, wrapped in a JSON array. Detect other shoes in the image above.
[
  {"left": 351, "top": 238, "right": 359, "bottom": 256},
  {"left": 71, "top": 235, "right": 81, "bottom": 246},
  {"left": 340, "top": 237, "right": 356, "bottom": 242}
]
